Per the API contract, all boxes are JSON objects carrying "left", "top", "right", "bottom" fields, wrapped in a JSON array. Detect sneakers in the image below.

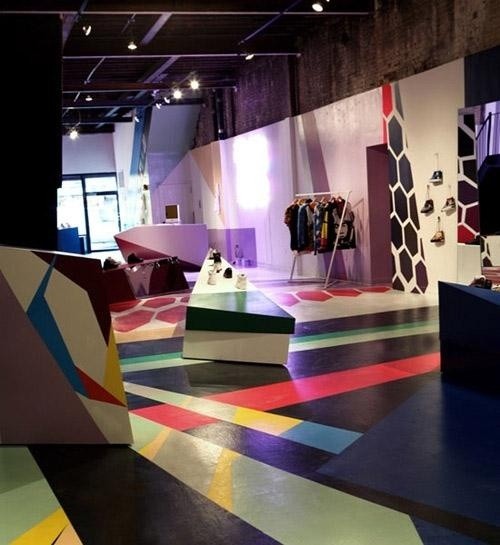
[
  {"left": 223, "top": 268, "right": 232, "bottom": 278},
  {"left": 441, "top": 197, "right": 456, "bottom": 212},
  {"left": 213, "top": 252, "right": 222, "bottom": 263},
  {"left": 429, "top": 171, "right": 443, "bottom": 183},
  {"left": 235, "top": 273, "right": 247, "bottom": 289},
  {"left": 420, "top": 199, "right": 434, "bottom": 213},
  {"left": 430, "top": 231, "right": 445, "bottom": 242},
  {"left": 209, "top": 249, "right": 216, "bottom": 259},
  {"left": 214, "top": 261, "right": 222, "bottom": 273},
  {"left": 208, "top": 269, "right": 216, "bottom": 285}
]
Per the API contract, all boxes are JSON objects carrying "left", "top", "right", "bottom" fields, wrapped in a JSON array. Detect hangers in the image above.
[{"left": 288, "top": 193, "right": 350, "bottom": 208}]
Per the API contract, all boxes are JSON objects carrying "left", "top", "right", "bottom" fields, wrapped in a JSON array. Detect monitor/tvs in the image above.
[{"left": 165, "top": 204, "right": 180, "bottom": 222}]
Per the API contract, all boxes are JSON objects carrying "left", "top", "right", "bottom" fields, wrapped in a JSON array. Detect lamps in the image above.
[
  {"left": 312, "top": 1, "right": 324, "bottom": 12},
  {"left": 84, "top": 93, "right": 94, "bottom": 102},
  {"left": 243, "top": 52, "right": 254, "bottom": 60},
  {"left": 128, "top": 41, "right": 137, "bottom": 50},
  {"left": 83, "top": 25, "right": 89, "bottom": 36},
  {"left": 133, "top": 114, "right": 143, "bottom": 123}
]
